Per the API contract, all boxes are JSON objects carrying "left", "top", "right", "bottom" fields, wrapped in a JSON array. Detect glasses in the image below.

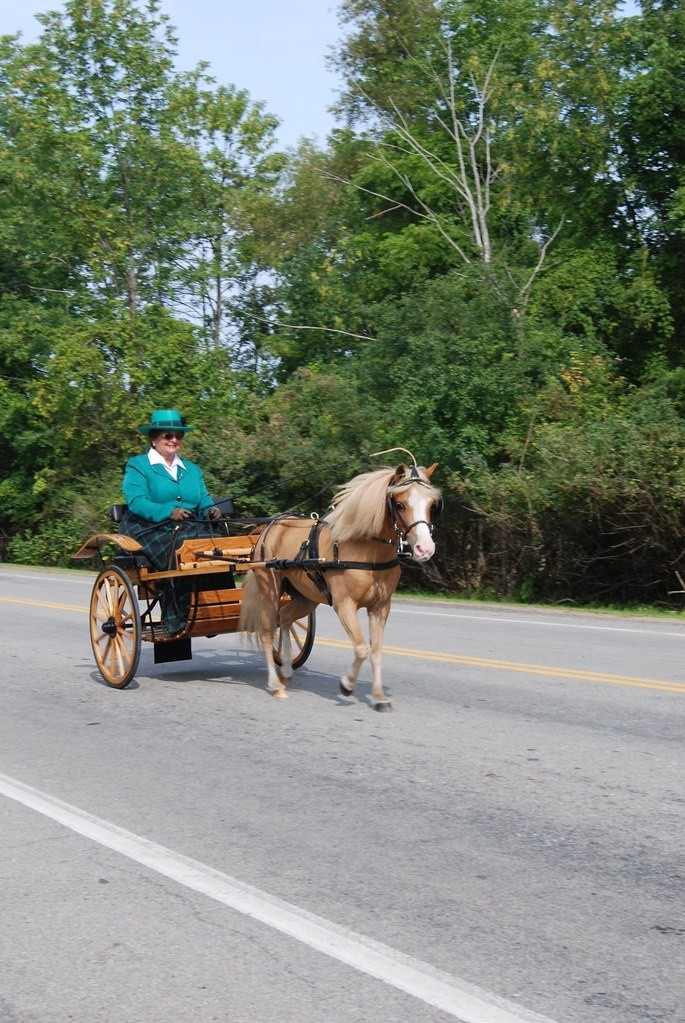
[{"left": 162, "top": 432, "right": 184, "bottom": 440}]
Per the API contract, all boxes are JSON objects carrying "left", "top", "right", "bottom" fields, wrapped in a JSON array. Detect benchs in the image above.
[{"left": 110, "top": 497, "right": 233, "bottom": 568}]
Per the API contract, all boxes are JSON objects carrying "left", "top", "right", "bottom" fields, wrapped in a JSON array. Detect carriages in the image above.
[{"left": 69, "top": 447, "right": 443, "bottom": 713}]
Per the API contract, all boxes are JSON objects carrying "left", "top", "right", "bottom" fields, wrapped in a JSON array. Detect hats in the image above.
[{"left": 138, "top": 409, "right": 193, "bottom": 435}]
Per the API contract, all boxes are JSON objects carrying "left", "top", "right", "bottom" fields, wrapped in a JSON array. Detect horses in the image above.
[{"left": 235, "top": 462, "right": 444, "bottom": 711}]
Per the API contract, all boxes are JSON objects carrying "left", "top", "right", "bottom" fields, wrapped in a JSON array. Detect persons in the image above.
[{"left": 119, "top": 409, "right": 236, "bottom": 635}]
